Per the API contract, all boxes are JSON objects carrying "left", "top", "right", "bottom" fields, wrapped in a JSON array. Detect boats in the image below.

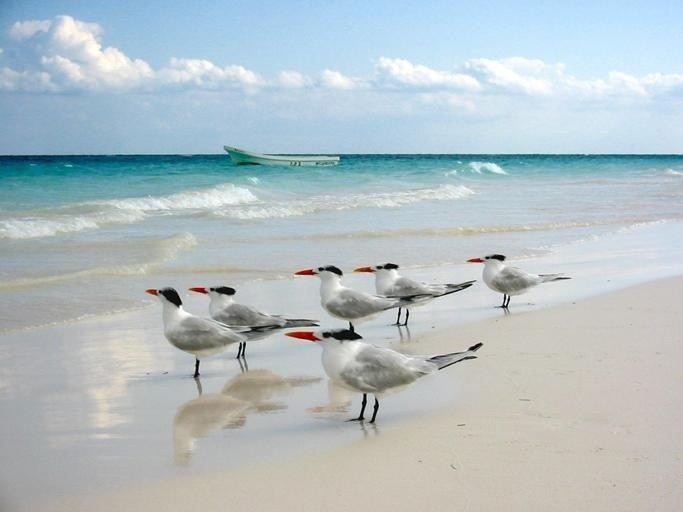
[{"left": 222, "top": 144, "right": 341, "bottom": 169}]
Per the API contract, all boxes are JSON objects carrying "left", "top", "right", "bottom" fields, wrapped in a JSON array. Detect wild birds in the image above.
[
  {"left": 145, "top": 288, "right": 282, "bottom": 378},
  {"left": 467, "top": 253, "right": 573, "bottom": 309},
  {"left": 294, "top": 265, "right": 432, "bottom": 331},
  {"left": 188, "top": 286, "right": 320, "bottom": 358},
  {"left": 285, "top": 329, "right": 483, "bottom": 424},
  {"left": 353, "top": 263, "right": 478, "bottom": 327}
]
